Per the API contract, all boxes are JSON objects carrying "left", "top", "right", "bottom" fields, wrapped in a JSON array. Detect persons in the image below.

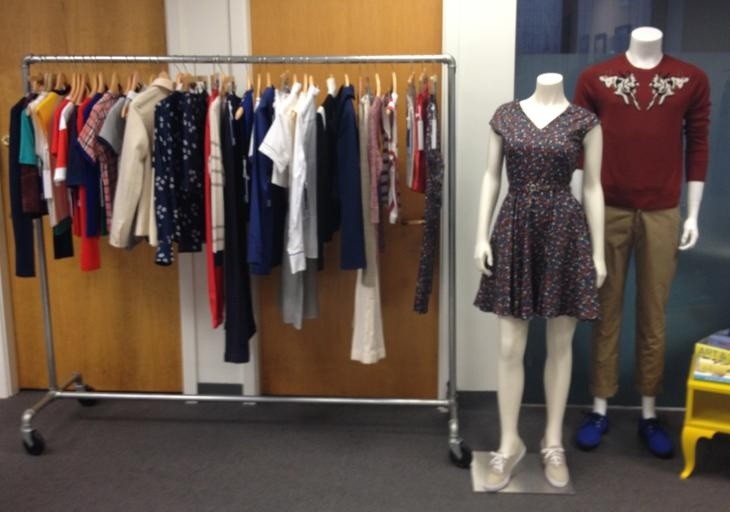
[
  {"left": 571, "top": 25, "right": 711, "bottom": 459},
  {"left": 472, "top": 69, "right": 608, "bottom": 493}
]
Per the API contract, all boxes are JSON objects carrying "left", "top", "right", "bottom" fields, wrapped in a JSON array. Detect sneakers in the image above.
[
  {"left": 640, "top": 420, "right": 674, "bottom": 457},
  {"left": 485, "top": 438, "right": 525, "bottom": 493},
  {"left": 540, "top": 440, "right": 568, "bottom": 487},
  {"left": 576, "top": 413, "right": 608, "bottom": 451}
]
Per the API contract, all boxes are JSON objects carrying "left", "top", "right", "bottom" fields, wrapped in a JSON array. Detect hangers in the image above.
[{"left": 18, "top": 50, "right": 445, "bottom": 125}]
